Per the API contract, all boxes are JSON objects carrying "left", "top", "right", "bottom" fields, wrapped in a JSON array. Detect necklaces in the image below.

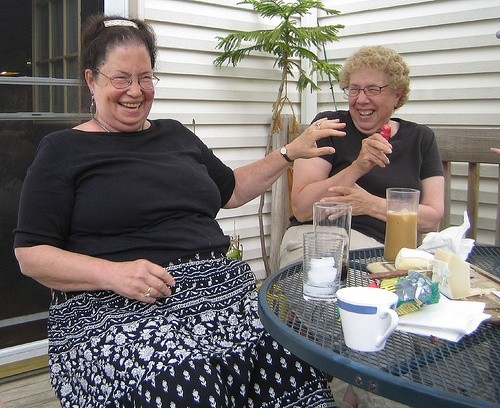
[{"left": 92, "top": 118, "right": 144, "bottom": 132}]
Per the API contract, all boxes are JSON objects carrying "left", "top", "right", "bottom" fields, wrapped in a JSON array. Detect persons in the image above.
[
  {"left": 13, "top": 16, "right": 347, "bottom": 408},
  {"left": 280, "top": 44, "right": 446, "bottom": 408}
]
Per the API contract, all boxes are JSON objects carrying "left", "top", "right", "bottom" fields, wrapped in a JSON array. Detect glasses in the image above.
[
  {"left": 342, "top": 84, "right": 391, "bottom": 97},
  {"left": 92, "top": 67, "right": 160, "bottom": 91}
]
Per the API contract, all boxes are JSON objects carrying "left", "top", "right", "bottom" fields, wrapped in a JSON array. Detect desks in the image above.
[{"left": 259, "top": 243, "right": 500, "bottom": 408}]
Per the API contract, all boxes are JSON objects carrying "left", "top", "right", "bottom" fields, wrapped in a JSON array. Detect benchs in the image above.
[{"left": 296, "top": 123, "right": 500, "bottom": 246}]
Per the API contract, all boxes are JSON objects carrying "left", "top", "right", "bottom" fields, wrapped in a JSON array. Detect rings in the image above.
[
  {"left": 144, "top": 288, "right": 151, "bottom": 297},
  {"left": 316, "top": 121, "right": 320, "bottom": 129}
]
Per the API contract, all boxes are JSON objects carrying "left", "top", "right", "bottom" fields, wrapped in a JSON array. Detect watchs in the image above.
[{"left": 280, "top": 145, "right": 294, "bottom": 162}]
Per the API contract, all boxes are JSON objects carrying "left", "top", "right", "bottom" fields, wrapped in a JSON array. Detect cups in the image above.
[
  {"left": 312, "top": 202, "right": 357, "bottom": 288},
  {"left": 335, "top": 287, "right": 399, "bottom": 352},
  {"left": 383, "top": 187, "right": 420, "bottom": 261},
  {"left": 301, "top": 231, "right": 344, "bottom": 302}
]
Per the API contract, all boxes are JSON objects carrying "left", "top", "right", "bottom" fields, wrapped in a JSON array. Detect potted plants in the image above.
[{"left": 213, "top": 0, "right": 345, "bottom": 324}]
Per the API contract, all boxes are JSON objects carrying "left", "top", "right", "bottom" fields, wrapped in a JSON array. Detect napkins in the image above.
[{"left": 398, "top": 301, "right": 492, "bottom": 344}]
